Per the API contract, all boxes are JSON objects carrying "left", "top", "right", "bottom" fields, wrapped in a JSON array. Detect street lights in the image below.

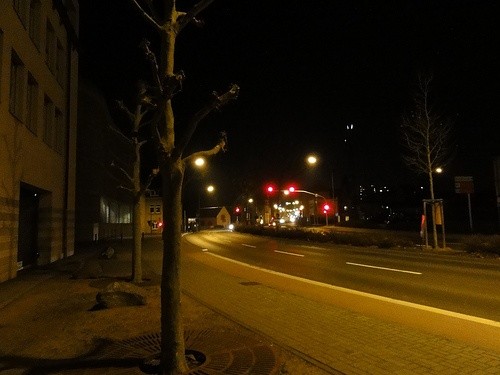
[
  {"left": 429, "top": 168, "right": 444, "bottom": 249},
  {"left": 308, "top": 154, "right": 336, "bottom": 228},
  {"left": 198, "top": 184, "right": 215, "bottom": 230}
]
